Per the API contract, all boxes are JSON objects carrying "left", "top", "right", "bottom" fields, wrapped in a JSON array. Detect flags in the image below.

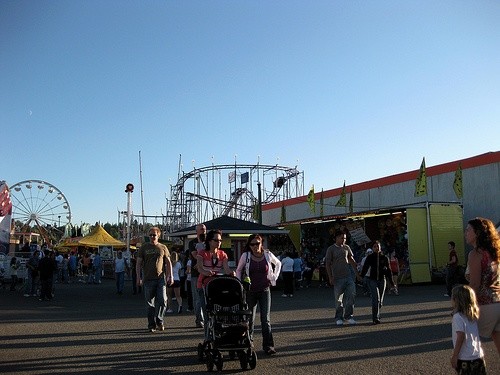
[
  {"left": 413, "top": 161, "right": 426, "bottom": 197},
  {"left": 307, "top": 189, "right": 324, "bottom": 217},
  {"left": 452, "top": 165, "right": 464, "bottom": 201},
  {"left": 336, "top": 185, "right": 353, "bottom": 213}
]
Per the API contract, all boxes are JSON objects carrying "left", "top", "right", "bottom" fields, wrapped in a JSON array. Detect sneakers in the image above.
[
  {"left": 344, "top": 319, "right": 356, "bottom": 324},
  {"left": 337, "top": 320, "right": 343, "bottom": 325}
]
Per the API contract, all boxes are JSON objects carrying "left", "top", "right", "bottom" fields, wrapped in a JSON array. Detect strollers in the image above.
[{"left": 197, "top": 273, "right": 258, "bottom": 372}]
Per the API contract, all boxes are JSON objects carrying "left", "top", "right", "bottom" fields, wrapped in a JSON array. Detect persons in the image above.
[
  {"left": 444, "top": 241, "right": 459, "bottom": 297},
  {"left": 163, "top": 245, "right": 194, "bottom": 314},
  {"left": 188, "top": 222, "right": 208, "bottom": 328},
  {"left": 52, "top": 246, "right": 103, "bottom": 284},
  {"left": 387, "top": 248, "right": 400, "bottom": 294},
  {"left": 135, "top": 226, "right": 173, "bottom": 332},
  {"left": 449, "top": 285, "right": 487, "bottom": 375},
  {"left": 325, "top": 231, "right": 362, "bottom": 324},
  {"left": 273, "top": 250, "right": 328, "bottom": 297},
  {"left": 112, "top": 250, "right": 128, "bottom": 294},
  {"left": 196, "top": 229, "right": 230, "bottom": 309},
  {"left": 360, "top": 248, "right": 372, "bottom": 296},
  {"left": 360, "top": 240, "right": 398, "bottom": 324},
  {"left": 9, "top": 243, "right": 57, "bottom": 304},
  {"left": 463, "top": 217, "right": 500, "bottom": 354},
  {"left": 195, "top": 233, "right": 206, "bottom": 252},
  {"left": 236, "top": 233, "right": 281, "bottom": 354}
]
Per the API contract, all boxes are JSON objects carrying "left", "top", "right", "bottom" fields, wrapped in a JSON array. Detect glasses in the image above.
[
  {"left": 212, "top": 239, "right": 223, "bottom": 244},
  {"left": 249, "top": 242, "right": 262, "bottom": 247},
  {"left": 149, "top": 233, "right": 159, "bottom": 237}
]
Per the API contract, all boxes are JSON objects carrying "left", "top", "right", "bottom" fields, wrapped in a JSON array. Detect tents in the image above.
[{"left": 77, "top": 224, "right": 128, "bottom": 261}]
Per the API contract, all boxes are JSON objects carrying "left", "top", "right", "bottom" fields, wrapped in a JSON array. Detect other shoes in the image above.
[
  {"left": 37, "top": 298, "right": 42, "bottom": 301},
  {"left": 290, "top": 295, "right": 293, "bottom": 297},
  {"left": 24, "top": 294, "right": 30, "bottom": 296},
  {"left": 166, "top": 309, "right": 173, "bottom": 313},
  {"left": 150, "top": 325, "right": 157, "bottom": 332},
  {"left": 186, "top": 309, "right": 192, "bottom": 312},
  {"left": 178, "top": 305, "right": 183, "bottom": 314},
  {"left": 250, "top": 340, "right": 254, "bottom": 347},
  {"left": 157, "top": 322, "right": 165, "bottom": 331},
  {"left": 282, "top": 294, "right": 288, "bottom": 297},
  {"left": 266, "top": 346, "right": 276, "bottom": 353},
  {"left": 373, "top": 318, "right": 380, "bottom": 324},
  {"left": 196, "top": 322, "right": 204, "bottom": 327},
  {"left": 10, "top": 288, "right": 17, "bottom": 291},
  {"left": 33, "top": 293, "right": 37, "bottom": 296}
]
